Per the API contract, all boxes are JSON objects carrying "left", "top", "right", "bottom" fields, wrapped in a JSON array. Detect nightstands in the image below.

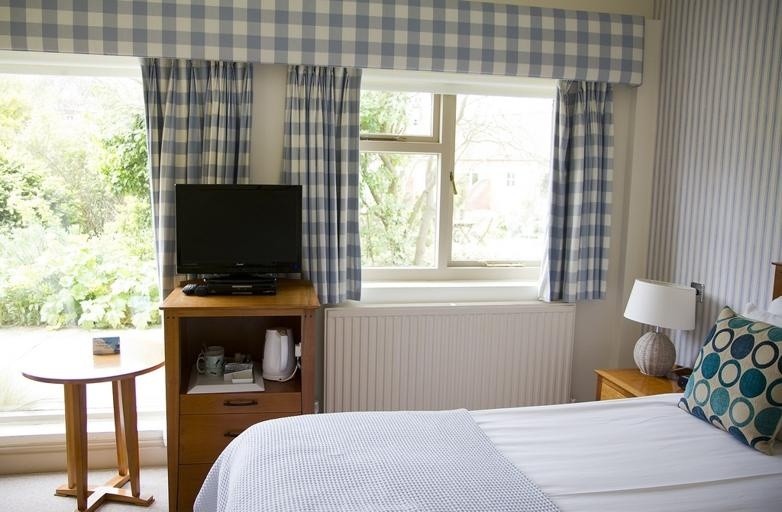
[{"left": 593, "top": 363, "right": 682, "bottom": 399}]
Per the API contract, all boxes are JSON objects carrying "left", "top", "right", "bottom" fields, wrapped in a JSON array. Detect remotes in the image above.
[{"left": 181, "top": 283, "right": 197, "bottom": 294}]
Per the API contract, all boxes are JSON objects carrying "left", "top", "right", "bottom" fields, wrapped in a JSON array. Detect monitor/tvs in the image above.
[{"left": 174, "top": 184, "right": 303, "bottom": 296}]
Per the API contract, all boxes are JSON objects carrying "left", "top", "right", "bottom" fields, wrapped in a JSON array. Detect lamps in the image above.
[{"left": 622, "top": 279, "right": 696, "bottom": 374}]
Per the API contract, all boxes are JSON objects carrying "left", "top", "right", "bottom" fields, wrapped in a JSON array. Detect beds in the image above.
[{"left": 221, "top": 261, "right": 782, "bottom": 512}]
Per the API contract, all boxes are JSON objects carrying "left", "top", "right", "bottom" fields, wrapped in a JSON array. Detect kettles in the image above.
[{"left": 262, "top": 326, "right": 296, "bottom": 382}]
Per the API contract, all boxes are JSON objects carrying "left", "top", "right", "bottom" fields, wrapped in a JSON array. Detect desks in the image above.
[{"left": 18, "top": 347, "right": 166, "bottom": 509}]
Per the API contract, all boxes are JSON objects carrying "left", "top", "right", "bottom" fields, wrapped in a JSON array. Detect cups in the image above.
[{"left": 193, "top": 346, "right": 224, "bottom": 377}]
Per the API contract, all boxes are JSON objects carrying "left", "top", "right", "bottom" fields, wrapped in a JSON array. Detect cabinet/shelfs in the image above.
[{"left": 163, "top": 280, "right": 318, "bottom": 510}]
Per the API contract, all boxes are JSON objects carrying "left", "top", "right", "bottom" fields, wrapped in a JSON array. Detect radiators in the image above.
[{"left": 322, "top": 299, "right": 573, "bottom": 409}]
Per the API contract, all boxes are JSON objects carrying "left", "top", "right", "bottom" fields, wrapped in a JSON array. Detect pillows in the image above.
[{"left": 675, "top": 309, "right": 782, "bottom": 456}]
